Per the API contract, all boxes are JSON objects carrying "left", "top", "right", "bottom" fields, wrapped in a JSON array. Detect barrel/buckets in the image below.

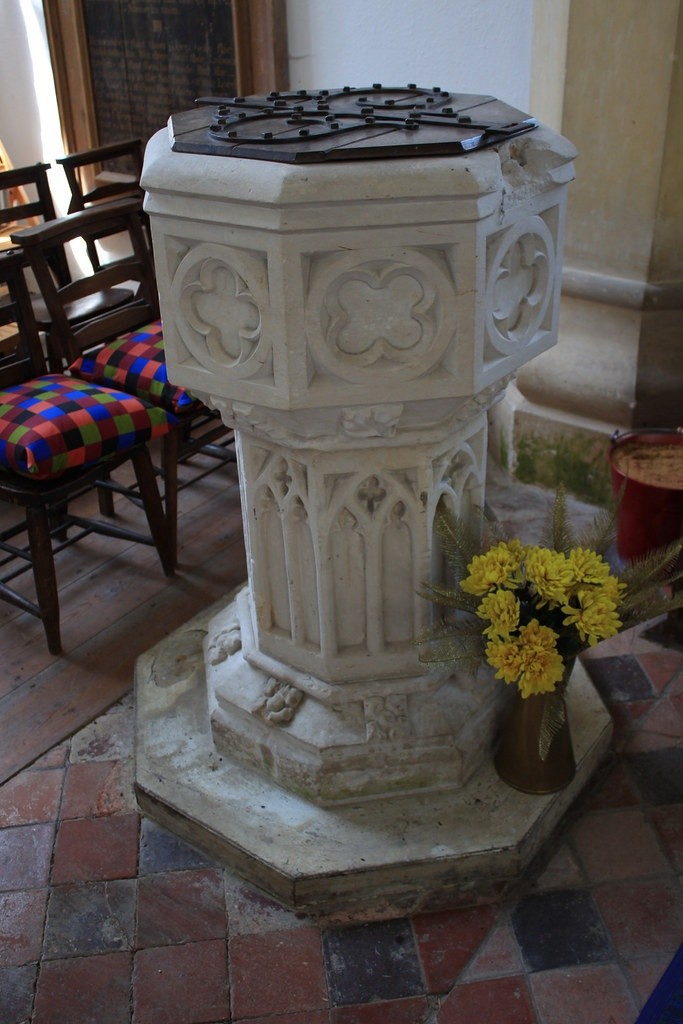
[{"left": 609, "top": 425, "right": 683, "bottom": 582}]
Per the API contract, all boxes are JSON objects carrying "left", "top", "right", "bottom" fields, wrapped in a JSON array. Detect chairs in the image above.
[{"left": 0, "top": 139, "right": 236, "bottom": 655}]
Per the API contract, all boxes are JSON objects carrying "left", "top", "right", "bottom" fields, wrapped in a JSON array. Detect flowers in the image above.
[{"left": 411, "top": 461, "right": 683, "bottom": 699}]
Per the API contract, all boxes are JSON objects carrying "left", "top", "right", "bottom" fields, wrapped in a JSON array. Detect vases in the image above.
[{"left": 494, "top": 675, "right": 576, "bottom": 795}]
